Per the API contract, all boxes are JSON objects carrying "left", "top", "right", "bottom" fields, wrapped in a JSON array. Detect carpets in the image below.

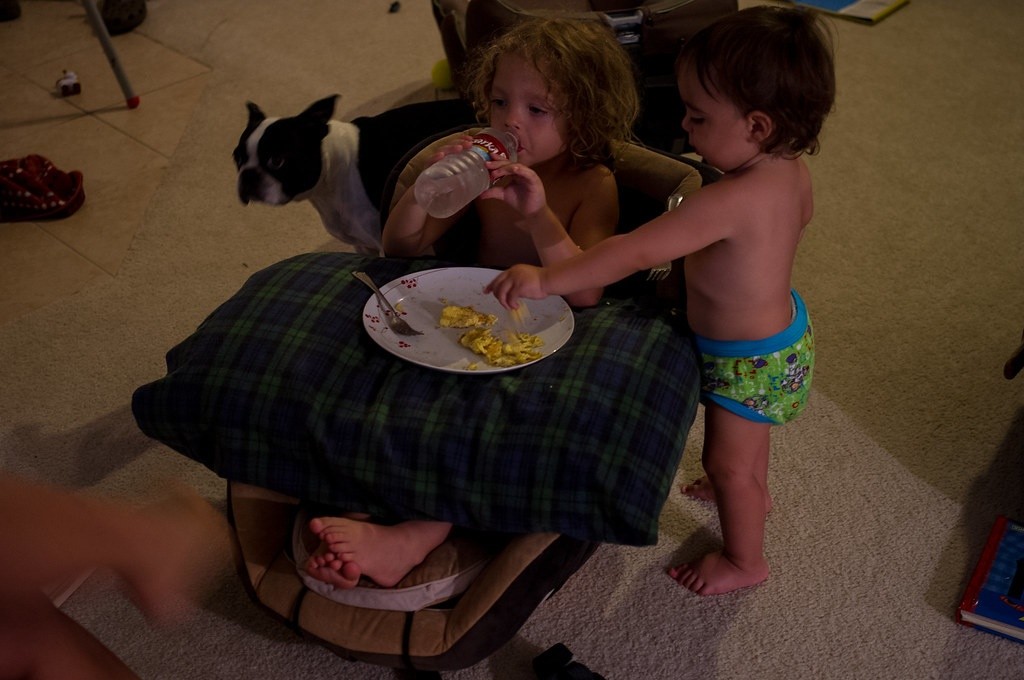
[{"left": 0, "top": 0, "right": 1024, "bottom": 680}]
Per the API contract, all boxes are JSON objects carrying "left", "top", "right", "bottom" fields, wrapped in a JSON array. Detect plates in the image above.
[{"left": 361, "top": 265, "right": 575, "bottom": 377}]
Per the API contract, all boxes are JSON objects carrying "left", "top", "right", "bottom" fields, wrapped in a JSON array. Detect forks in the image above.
[
  {"left": 640, "top": 194, "right": 689, "bottom": 287},
  {"left": 351, "top": 269, "right": 425, "bottom": 339}
]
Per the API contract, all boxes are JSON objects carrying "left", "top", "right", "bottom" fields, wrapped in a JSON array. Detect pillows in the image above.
[{"left": 130, "top": 250, "right": 702, "bottom": 547}]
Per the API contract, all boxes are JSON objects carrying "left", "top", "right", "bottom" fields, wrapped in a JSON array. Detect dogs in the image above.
[{"left": 231, "top": 94, "right": 485, "bottom": 255}]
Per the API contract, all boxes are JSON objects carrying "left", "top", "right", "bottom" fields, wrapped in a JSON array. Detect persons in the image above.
[
  {"left": 485, "top": 4, "right": 836, "bottom": 595},
  {"left": 0, "top": 466, "right": 241, "bottom": 680},
  {"left": 304, "top": 15, "right": 639, "bottom": 589}
]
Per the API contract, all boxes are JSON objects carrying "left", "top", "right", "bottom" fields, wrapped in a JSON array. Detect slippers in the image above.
[{"left": 0, "top": 154, "right": 86, "bottom": 220}]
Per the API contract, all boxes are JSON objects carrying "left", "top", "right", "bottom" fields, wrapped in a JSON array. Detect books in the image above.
[{"left": 955, "top": 514, "right": 1024, "bottom": 645}]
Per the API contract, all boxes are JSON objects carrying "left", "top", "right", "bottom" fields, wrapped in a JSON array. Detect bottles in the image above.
[{"left": 413, "top": 127, "right": 521, "bottom": 220}]
